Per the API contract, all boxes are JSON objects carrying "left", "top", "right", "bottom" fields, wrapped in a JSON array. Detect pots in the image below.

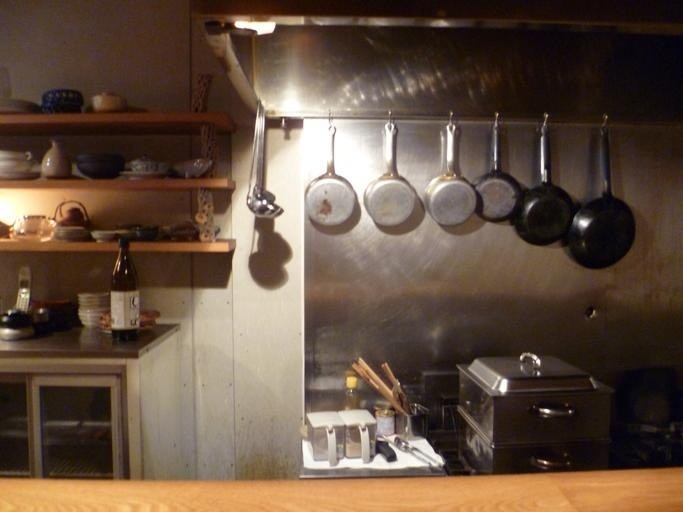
[
  {"left": 304, "top": 123, "right": 358, "bottom": 228},
  {"left": 363, "top": 121, "right": 417, "bottom": 228},
  {"left": 423, "top": 121, "right": 478, "bottom": 228},
  {"left": 472, "top": 120, "right": 522, "bottom": 223},
  {"left": 514, "top": 122, "right": 574, "bottom": 247},
  {"left": 570, "top": 125, "right": 636, "bottom": 270}
]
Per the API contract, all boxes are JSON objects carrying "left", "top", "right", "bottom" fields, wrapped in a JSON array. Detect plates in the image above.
[
  {"left": 120, "top": 172, "right": 172, "bottom": 181},
  {"left": 0, "top": 97, "right": 43, "bottom": 120},
  {"left": 0, "top": 172, "right": 40, "bottom": 181}
]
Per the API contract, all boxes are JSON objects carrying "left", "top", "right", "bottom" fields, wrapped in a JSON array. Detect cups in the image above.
[{"left": 128, "top": 156, "right": 160, "bottom": 172}]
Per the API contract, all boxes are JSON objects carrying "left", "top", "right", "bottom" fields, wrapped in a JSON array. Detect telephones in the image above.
[{"left": 0, "top": 266, "right": 37, "bottom": 340}]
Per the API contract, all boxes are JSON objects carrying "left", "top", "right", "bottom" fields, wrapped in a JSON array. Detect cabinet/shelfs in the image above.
[
  {"left": 0, "top": 324, "right": 183, "bottom": 476},
  {"left": 0, "top": 110, "right": 234, "bottom": 255}
]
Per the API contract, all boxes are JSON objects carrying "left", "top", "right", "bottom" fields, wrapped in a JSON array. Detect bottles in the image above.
[
  {"left": 109, "top": 237, "right": 141, "bottom": 340},
  {"left": 40, "top": 137, "right": 73, "bottom": 180},
  {"left": 341, "top": 377, "right": 361, "bottom": 411}
]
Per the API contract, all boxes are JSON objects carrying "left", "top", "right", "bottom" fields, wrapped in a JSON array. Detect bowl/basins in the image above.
[
  {"left": 76, "top": 152, "right": 126, "bottom": 181},
  {"left": 1, "top": 290, "right": 110, "bottom": 340},
  {"left": 0, "top": 150, "right": 34, "bottom": 173},
  {"left": 91, "top": 91, "right": 127, "bottom": 112},
  {"left": 0, "top": 199, "right": 199, "bottom": 243}
]
